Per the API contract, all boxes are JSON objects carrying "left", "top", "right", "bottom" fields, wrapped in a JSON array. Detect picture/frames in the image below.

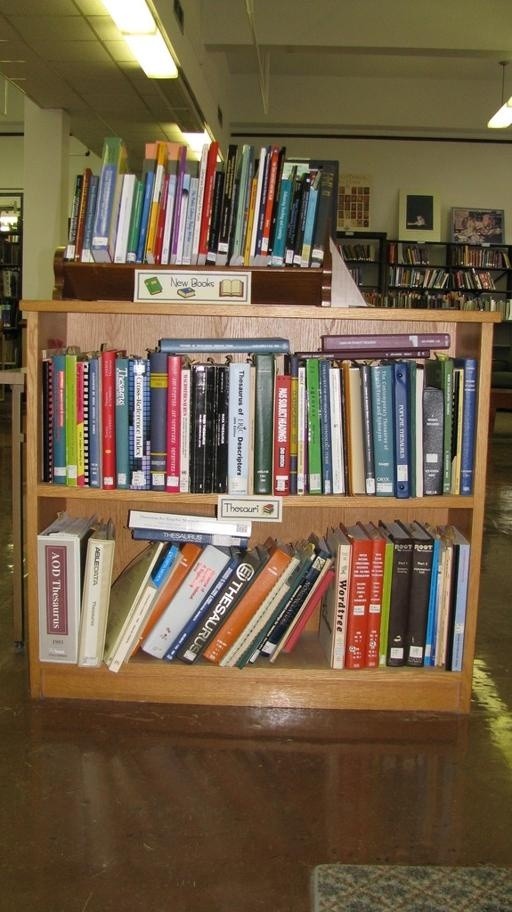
[
  {"left": 448, "top": 206, "right": 506, "bottom": 247},
  {"left": 398, "top": 187, "right": 442, "bottom": 243}
]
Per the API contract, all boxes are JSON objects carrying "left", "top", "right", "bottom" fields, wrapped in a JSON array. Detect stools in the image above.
[{"left": 486, "top": 359, "right": 512, "bottom": 465}]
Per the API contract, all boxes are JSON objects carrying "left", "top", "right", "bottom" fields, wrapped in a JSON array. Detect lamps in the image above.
[
  {"left": 106, "top": 0, "right": 186, "bottom": 81},
  {"left": 486, "top": 60, "right": 512, "bottom": 131}
]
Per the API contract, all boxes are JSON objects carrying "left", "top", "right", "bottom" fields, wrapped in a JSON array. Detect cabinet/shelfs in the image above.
[
  {"left": 333, "top": 231, "right": 388, "bottom": 306},
  {"left": 1, "top": 190, "right": 28, "bottom": 365},
  {"left": 15, "top": 298, "right": 506, "bottom": 716},
  {"left": 447, "top": 242, "right": 512, "bottom": 322},
  {"left": 386, "top": 238, "right": 448, "bottom": 309}
]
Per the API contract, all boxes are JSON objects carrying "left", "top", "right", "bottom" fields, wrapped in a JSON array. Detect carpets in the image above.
[{"left": 304, "top": 855, "right": 509, "bottom": 912}]
